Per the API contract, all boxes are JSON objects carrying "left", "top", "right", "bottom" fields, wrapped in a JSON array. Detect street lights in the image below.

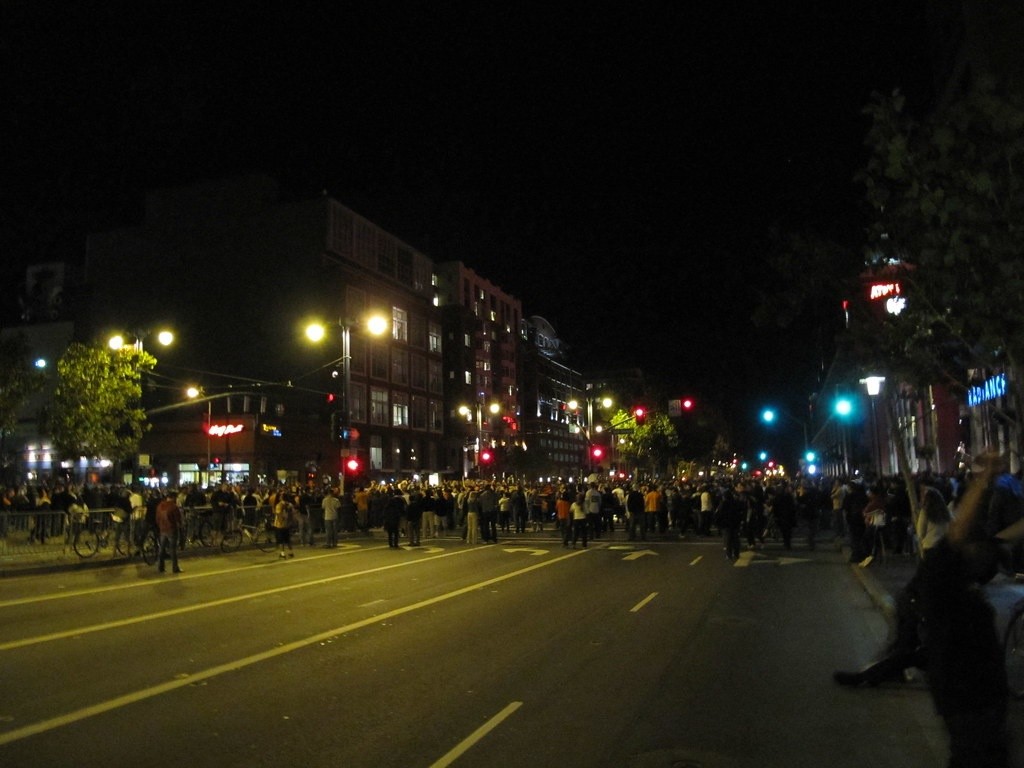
[
  {"left": 459, "top": 401, "right": 502, "bottom": 481},
  {"left": 110, "top": 329, "right": 174, "bottom": 411},
  {"left": 567, "top": 396, "right": 613, "bottom": 471},
  {"left": 304, "top": 310, "right": 391, "bottom": 496}
]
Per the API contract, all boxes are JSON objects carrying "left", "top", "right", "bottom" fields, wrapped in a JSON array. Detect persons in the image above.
[
  {"left": 434, "top": 490, "right": 449, "bottom": 538},
  {"left": 466, "top": 493, "right": 479, "bottom": 545},
  {"left": 321, "top": 490, "right": 344, "bottom": 549},
  {"left": 556, "top": 492, "right": 571, "bottom": 547},
  {"left": 354, "top": 486, "right": 368, "bottom": 529},
  {"left": 422, "top": 490, "right": 435, "bottom": 538},
  {"left": 481, "top": 490, "right": 497, "bottom": 544},
  {"left": 627, "top": 484, "right": 646, "bottom": 541},
  {"left": 643, "top": 485, "right": 660, "bottom": 533},
  {"left": 273, "top": 492, "right": 294, "bottom": 558},
  {"left": 342, "top": 489, "right": 354, "bottom": 532},
  {"left": 511, "top": 487, "right": 527, "bottom": 534},
  {"left": 406, "top": 496, "right": 421, "bottom": 547},
  {"left": 384, "top": 490, "right": 407, "bottom": 548},
  {"left": 499, "top": 493, "right": 511, "bottom": 533},
  {"left": 701, "top": 487, "right": 713, "bottom": 535},
  {"left": 570, "top": 494, "right": 589, "bottom": 549},
  {"left": 0, "top": 451, "right": 1024, "bottom": 768},
  {"left": 156, "top": 495, "right": 183, "bottom": 574}
]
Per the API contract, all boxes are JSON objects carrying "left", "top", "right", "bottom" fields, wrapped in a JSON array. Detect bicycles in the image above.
[
  {"left": 139, "top": 509, "right": 217, "bottom": 567},
  {"left": 865, "top": 503, "right": 892, "bottom": 568},
  {"left": 219, "top": 515, "right": 279, "bottom": 554},
  {"left": 72, "top": 520, "right": 136, "bottom": 559}
]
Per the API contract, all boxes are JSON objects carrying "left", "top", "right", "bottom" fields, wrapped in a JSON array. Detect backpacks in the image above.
[{"left": 589, "top": 496, "right": 600, "bottom": 514}]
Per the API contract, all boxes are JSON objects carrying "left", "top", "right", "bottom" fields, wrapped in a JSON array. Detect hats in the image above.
[{"left": 632, "top": 484, "right": 640, "bottom": 491}]
